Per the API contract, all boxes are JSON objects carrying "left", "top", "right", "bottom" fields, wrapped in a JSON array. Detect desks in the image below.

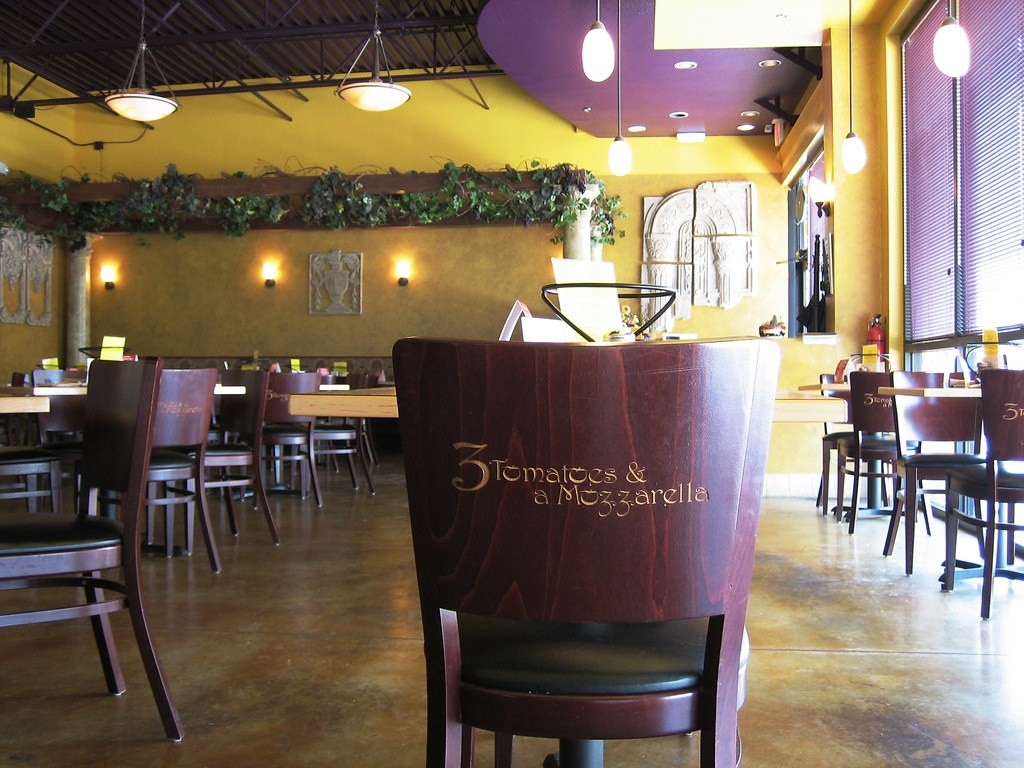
[
  {"left": 797, "top": 384, "right": 905, "bottom": 522},
  {"left": 286, "top": 386, "right": 399, "bottom": 418},
  {"left": 0, "top": 396, "right": 50, "bottom": 414},
  {"left": 0, "top": 385, "right": 245, "bottom": 519},
  {"left": 877, "top": 386, "right": 1024, "bottom": 590}
]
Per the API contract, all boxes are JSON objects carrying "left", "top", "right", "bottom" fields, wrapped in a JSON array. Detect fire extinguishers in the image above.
[{"left": 866, "top": 314, "right": 885, "bottom": 364}]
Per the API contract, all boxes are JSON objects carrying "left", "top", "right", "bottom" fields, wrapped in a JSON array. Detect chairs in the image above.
[{"left": 0, "top": 336, "right": 1024, "bottom": 768}]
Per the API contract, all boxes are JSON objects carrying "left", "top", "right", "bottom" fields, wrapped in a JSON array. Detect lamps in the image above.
[
  {"left": 104, "top": 0, "right": 178, "bottom": 122},
  {"left": 265, "top": 279, "right": 275, "bottom": 287},
  {"left": 608, "top": 0, "right": 631, "bottom": 177},
  {"left": 335, "top": 0, "right": 412, "bottom": 113},
  {"left": 399, "top": 278, "right": 408, "bottom": 286},
  {"left": 841, "top": 0, "right": 867, "bottom": 174},
  {"left": 933, "top": 0, "right": 970, "bottom": 77},
  {"left": 104, "top": 282, "right": 115, "bottom": 290},
  {"left": 582, "top": 0, "right": 615, "bottom": 82}
]
